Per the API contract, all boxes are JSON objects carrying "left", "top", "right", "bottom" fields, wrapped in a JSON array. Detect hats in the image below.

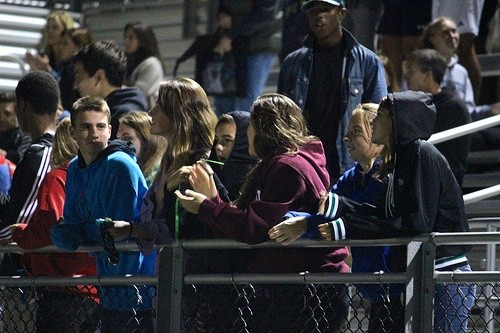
[{"left": 301, "top": 0, "right": 345, "bottom": 11}]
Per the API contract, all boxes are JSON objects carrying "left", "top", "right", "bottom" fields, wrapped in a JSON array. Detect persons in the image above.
[
  {"left": 278, "top": 0, "right": 388, "bottom": 183},
  {"left": 174, "top": 93, "right": 351, "bottom": 332},
  {"left": 402, "top": 48, "right": 473, "bottom": 192},
  {"left": 316, "top": 89, "right": 476, "bottom": 332},
  {"left": 267, "top": 102, "right": 409, "bottom": 333},
  {"left": 163, "top": 109, "right": 258, "bottom": 333},
  {"left": 280, "top": 0, "right": 499, "bottom": 115},
  {"left": 201, "top": 0, "right": 278, "bottom": 114},
  {"left": 0, "top": 8, "right": 219, "bottom": 332}
]
